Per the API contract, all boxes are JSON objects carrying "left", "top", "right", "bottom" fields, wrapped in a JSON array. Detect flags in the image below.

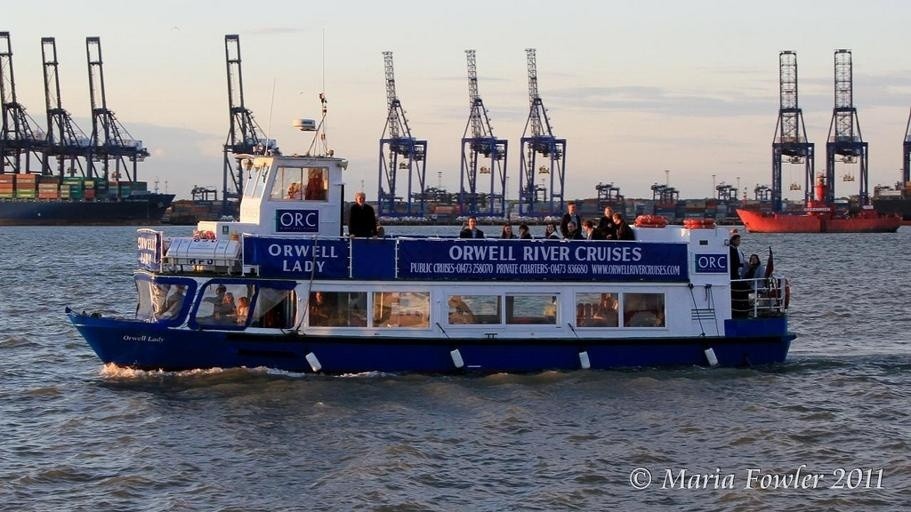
[{"left": 765, "top": 248, "right": 773, "bottom": 278}]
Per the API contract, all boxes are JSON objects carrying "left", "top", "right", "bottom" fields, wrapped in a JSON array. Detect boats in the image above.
[
  {"left": 65, "top": 154, "right": 801, "bottom": 376},
  {"left": 0, "top": 166, "right": 175, "bottom": 226},
  {"left": 736, "top": 171, "right": 905, "bottom": 234}
]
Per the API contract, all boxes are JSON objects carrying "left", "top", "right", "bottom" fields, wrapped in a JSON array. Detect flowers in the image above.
[{"left": 191, "top": 229, "right": 215, "bottom": 239}]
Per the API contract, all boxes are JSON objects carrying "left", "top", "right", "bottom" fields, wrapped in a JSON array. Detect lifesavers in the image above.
[{"left": 779, "top": 277, "right": 790, "bottom": 309}]
